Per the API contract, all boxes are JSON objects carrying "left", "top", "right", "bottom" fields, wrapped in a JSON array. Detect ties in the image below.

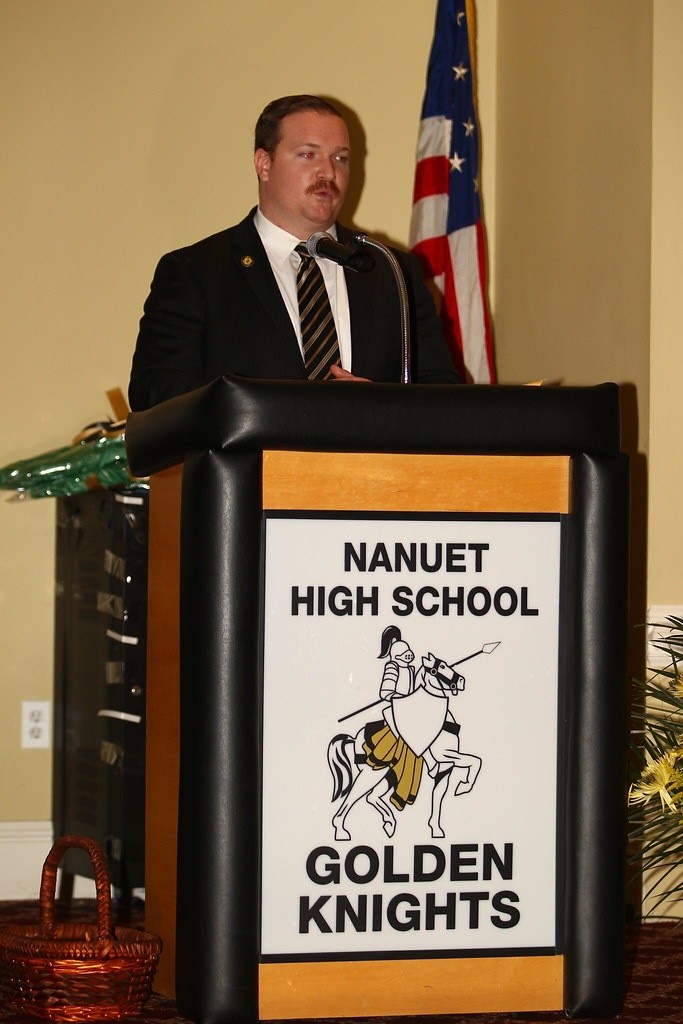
[{"left": 294, "top": 242, "right": 343, "bottom": 381}]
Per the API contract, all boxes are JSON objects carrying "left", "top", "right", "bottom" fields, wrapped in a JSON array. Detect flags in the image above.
[{"left": 409, "top": 0, "right": 498, "bottom": 382}]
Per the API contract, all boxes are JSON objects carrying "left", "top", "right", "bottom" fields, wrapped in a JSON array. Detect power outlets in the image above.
[{"left": 21, "top": 701, "right": 52, "bottom": 748}]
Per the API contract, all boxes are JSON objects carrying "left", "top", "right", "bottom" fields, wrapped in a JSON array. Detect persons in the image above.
[{"left": 128, "top": 93, "right": 469, "bottom": 412}]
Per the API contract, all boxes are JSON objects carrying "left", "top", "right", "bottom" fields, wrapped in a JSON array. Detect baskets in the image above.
[{"left": 0, "top": 833, "right": 161, "bottom": 1024}]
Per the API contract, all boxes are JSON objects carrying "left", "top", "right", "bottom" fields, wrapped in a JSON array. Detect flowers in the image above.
[{"left": 630, "top": 616, "right": 683, "bottom": 932}]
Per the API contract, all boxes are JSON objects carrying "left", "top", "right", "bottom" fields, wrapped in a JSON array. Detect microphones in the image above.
[{"left": 306, "top": 231, "right": 365, "bottom": 274}]
